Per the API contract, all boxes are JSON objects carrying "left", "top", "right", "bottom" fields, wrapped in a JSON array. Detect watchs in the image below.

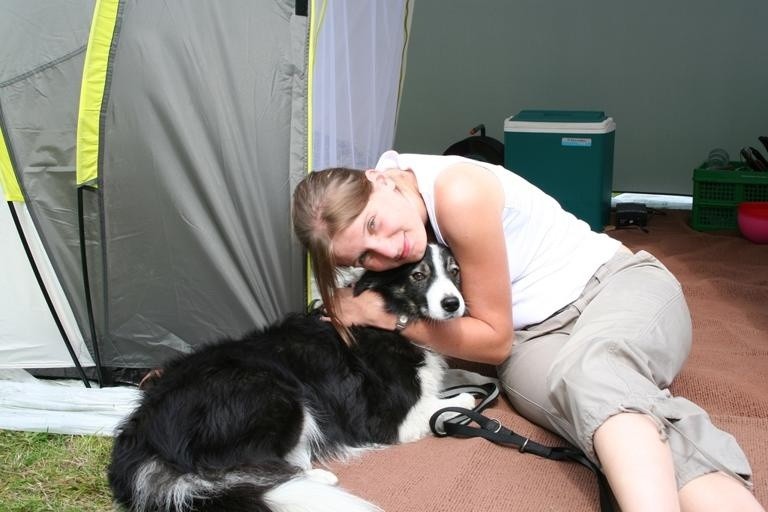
[{"left": 392, "top": 307, "right": 416, "bottom": 337}]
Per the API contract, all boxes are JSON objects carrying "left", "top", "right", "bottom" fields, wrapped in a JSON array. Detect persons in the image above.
[{"left": 291, "top": 148, "right": 768, "bottom": 510}]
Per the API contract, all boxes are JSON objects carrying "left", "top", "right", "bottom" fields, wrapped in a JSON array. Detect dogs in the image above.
[{"left": 105, "top": 237, "right": 475, "bottom": 512}]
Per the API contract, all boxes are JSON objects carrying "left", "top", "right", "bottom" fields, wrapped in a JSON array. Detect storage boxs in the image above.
[{"left": 502, "top": 105, "right": 768, "bottom": 242}]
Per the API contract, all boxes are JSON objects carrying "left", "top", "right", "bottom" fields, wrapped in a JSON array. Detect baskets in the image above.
[{"left": 687, "top": 159, "right": 767, "bottom": 233}]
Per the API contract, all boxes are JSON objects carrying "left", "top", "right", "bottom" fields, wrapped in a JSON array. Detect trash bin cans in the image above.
[{"left": 501, "top": 106, "right": 616, "bottom": 233}]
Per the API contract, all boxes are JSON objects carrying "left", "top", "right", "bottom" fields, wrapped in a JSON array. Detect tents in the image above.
[{"left": 0, "top": 0, "right": 414, "bottom": 441}]
